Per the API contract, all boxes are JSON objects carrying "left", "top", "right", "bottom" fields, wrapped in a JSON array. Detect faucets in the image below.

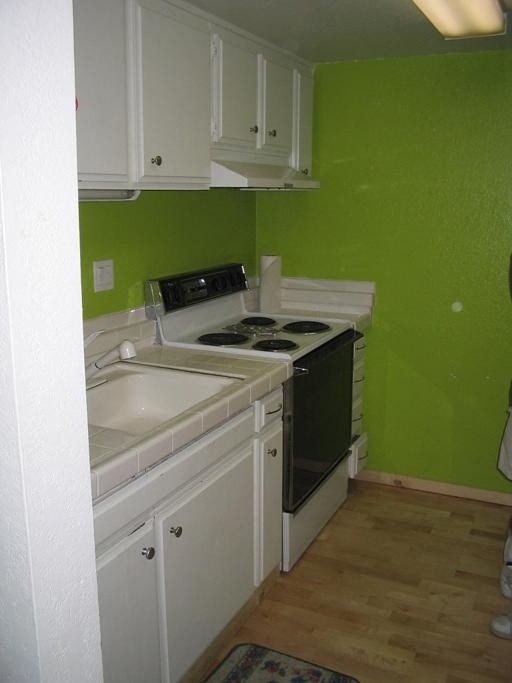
[{"left": 83, "top": 329, "right": 137, "bottom": 381}]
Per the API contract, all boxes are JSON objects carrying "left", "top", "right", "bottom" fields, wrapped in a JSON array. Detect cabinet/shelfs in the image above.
[
  {"left": 254, "top": 386, "right": 283, "bottom": 586},
  {"left": 292, "top": 50, "right": 314, "bottom": 178},
  {"left": 94, "top": 437, "right": 253, "bottom": 683},
  {"left": 73, "top": 0, "right": 128, "bottom": 184},
  {"left": 348, "top": 338, "right": 368, "bottom": 479},
  {"left": 126, "top": 0, "right": 211, "bottom": 190},
  {"left": 210, "top": 10, "right": 291, "bottom": 165}
]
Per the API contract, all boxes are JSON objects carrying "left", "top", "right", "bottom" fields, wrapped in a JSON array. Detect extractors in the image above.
[{"left": 210, "top": 161, "right": 320, "bottom": 195}]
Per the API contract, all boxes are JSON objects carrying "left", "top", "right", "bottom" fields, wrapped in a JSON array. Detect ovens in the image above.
[{"left": 281, "top": 327, "right": 365, "bottom": 514}]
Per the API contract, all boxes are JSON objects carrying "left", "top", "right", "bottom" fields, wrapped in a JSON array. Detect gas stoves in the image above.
[{"left": 170, "top": 311, "right": 352, "bottom": 363}]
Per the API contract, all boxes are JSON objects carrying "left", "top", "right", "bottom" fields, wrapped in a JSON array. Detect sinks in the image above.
[{"left": 87, "top": 361, "right": 251, "bottom": 441}]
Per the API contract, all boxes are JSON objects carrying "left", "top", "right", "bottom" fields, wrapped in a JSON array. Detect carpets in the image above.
[{"left": 204, "top": 643, "right": 359, "bottom": 683}]
